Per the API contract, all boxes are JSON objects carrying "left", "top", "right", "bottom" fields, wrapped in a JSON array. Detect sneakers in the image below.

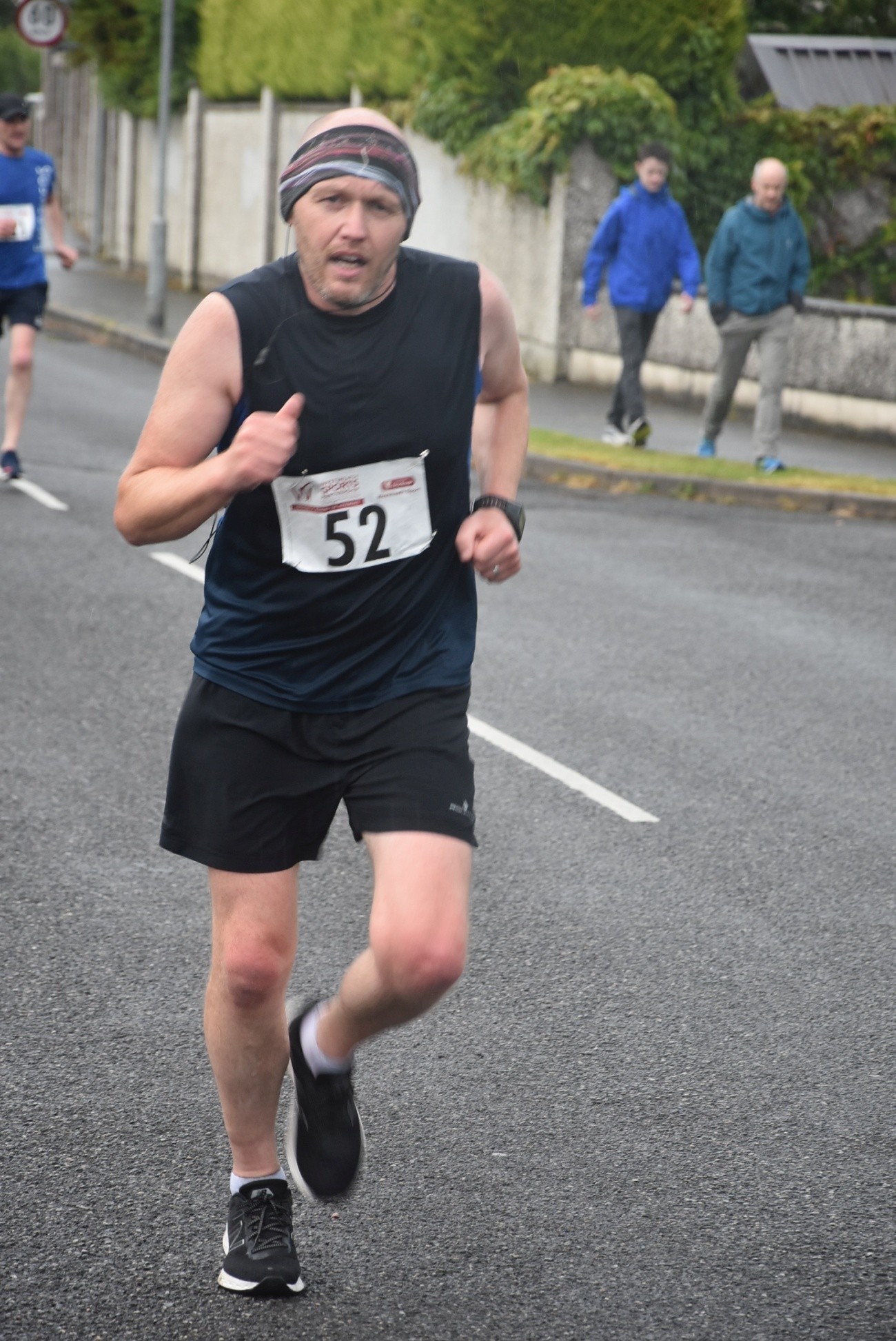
[
  {"left": 218, "top": 1180, "right": 305, "bottom": 1298},
  {"left": 286, "top": 996, "right": 369, "bottom": 1203}
]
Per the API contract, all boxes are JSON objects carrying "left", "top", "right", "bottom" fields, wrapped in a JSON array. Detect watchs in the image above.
[{"left": 472, "top": 496, "right": 528, "bottom": 543}]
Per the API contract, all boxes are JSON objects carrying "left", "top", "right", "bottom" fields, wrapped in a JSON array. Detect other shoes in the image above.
[
  {"left": 601, "top": 424, "right": 636, "bottom": 447},
  {"left": 0, "top": 450, "right": 24, "bottom": 484},
  {"left": 697, "top": 438, "right": 715, "bottom": 458},
  {"left": 629, "top": 417, "right": 651, "bottom": 446},
  {"left": 753, "top": 454, "right": 785, "bottom": 474}
]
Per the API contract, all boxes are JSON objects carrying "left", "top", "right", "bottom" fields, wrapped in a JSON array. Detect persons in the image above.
[
  {"left": 111, "top": 106, "right": 529, "bottom": 1293},
  {"left": 0, "top": 94, "right": 78, "bottom": 477},
  {"left": 700, "top": 156, "right": 812, "bottom": 470},
  {"left": 579, "top": 144, "right": 703, "bottom": 446}
]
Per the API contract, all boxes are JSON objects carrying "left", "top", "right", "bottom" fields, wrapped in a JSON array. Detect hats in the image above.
[{"left": 0, "top": 95, "right": 28, "bottom": 121}]
